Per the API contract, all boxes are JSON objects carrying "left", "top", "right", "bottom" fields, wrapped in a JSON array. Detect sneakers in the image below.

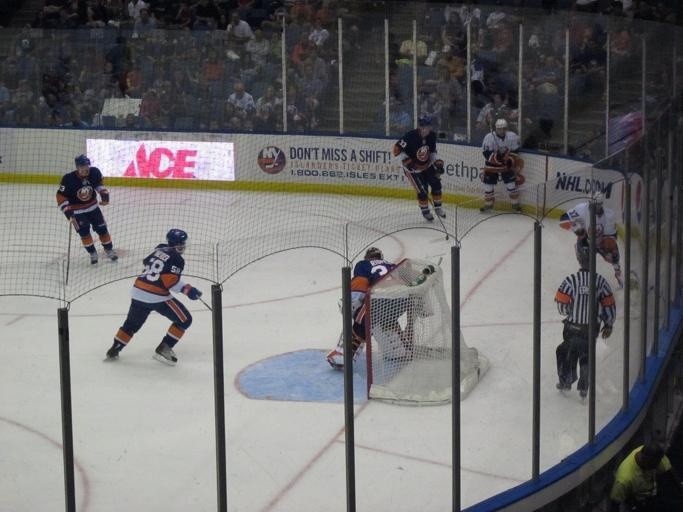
[
  {"left": 104, "top": 248, "right": 118, "bottom": 260},
  {"left": 615, "top": 273, "right": 623, "bottom": 288},
  {"left": 580, "top": 390, "right": 587, "bottom": 397},
  {"left": 512, "top": 204, "right": 521, "bottom": 210},
  {"left": 480, "top": 204, "right": 494, "bottom": 211},
  {"left": 90, "top": 250, "right": 97, "bottom": 264},
  {"left": 556, "top": 383, "right": 571, "bottom": 390}
]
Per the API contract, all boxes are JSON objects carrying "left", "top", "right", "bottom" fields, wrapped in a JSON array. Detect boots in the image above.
[
  {"left": 421, "top": 207, "right": 433, "bottom": 220},
  {"left": 107, "top": 338, "right": 125, "bottom": 356},
  {"left": 434, "top": 203, "right": 446, "bottom": 217},
  {"left": 156, "top": 336, "right": 177, "bottom": 362}
]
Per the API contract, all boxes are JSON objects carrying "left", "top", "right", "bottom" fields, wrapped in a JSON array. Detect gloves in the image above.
[
  {"left": 601, "top": 325, "right": 612, "bottom": 338},
  {"left": 575, "top": 228, "right": 588, "bottom": 245},
  {"left": 181, "top": 284, "right": 202, "bottom": 300},
  {"left": 402, "top": 158, "right": 414, "bottom": 168},
  {"left": 496, "top": 147, "right": 510, "bottom": 161},
  {"left": 100, "top": 189, "right": 109, "bottom": 205},
  {"left": 435, "top": 161, "right": 444, "bottom": 174},
  {"left": 61, "top": 204, "right": 74, "bottom": 219}
]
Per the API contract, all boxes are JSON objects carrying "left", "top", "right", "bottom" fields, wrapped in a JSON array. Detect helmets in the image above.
[
  {"left": 365, "top": 248, "right": 383, "bottom": 261},
  {"left": 495, "top": 119, "right": 507, "bottom": 128},
  {"left": 577, "top": 247, "right": 589, "bottom": 264},
  {"left": 418, "top": 115, "right": 432, "bottom": 124},
  {"left": 167, "top": 229, "right": 187, "bottom": 242},
  {"left": 75, "top": 155, "right": 90, "bottom": 169},
  {"left": 588, "top": 191, "right": 603, "bottom": 204}
]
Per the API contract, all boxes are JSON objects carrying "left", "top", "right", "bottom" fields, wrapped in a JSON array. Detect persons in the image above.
[
  {"left": 393, "top": 115, "right": 447, "bottom": 220},
  {"left": 479, "top": 116, "right": 523, "bottom": 215},
  {"left": 0, "top": 0, "right": 362, "bottom": 131},
  {"left": 393, "top": 1, "right": 651, "bottom": 149},
  {"left": 554, "top": 247, "right": 616, "bottom": 398},
  {"left": 558, "top": 192, "right": 624, "bottom": 287},
  {"left": 329, "top": 246, "right": 418, "bottom": 367},
  {"left": 57, "top": 154, "right": 118, "bottom": 263},
  {"left": 108, "top": 228, "right": 202, "bottom": 362}
]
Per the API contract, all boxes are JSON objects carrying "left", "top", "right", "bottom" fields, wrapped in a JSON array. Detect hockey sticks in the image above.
[
  {"left": 489, "top": 121, "right": 501, "bottom": 149},
  {"left": 413, "top": 168, "right": 450, "bottom": 240},
  {"left": 66, "top": 216, "right": 72, "bottom": 284}
]
